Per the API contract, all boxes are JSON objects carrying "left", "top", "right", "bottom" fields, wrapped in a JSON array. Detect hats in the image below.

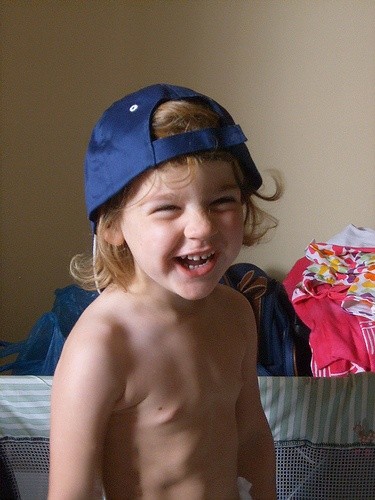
[{"left": 84, "top": 83, "right": 262, "bottom": 235}]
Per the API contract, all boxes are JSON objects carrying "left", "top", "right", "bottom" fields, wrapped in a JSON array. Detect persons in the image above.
[{"left": 46, "top": 83, "right": 279, "bottom": 499}]
